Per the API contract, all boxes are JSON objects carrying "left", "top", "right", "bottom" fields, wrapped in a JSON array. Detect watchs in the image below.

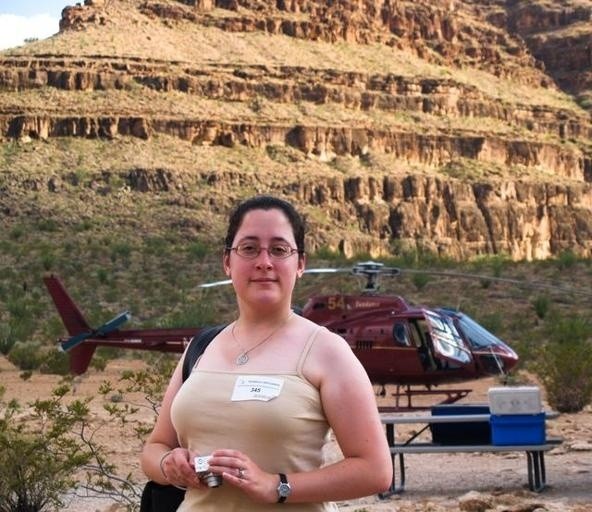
[{"left": 276, "top": 471, "right": 291, "bottom": 504}]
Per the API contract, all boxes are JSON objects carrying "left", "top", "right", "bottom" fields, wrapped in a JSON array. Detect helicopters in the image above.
[{"left": 40, "top": 254, "right": 529, "bottom": 418}]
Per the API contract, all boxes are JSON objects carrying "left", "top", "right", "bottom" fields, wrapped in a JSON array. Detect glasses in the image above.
[{"left": 227, "top": 241, "right": 298, "bottom": 259}]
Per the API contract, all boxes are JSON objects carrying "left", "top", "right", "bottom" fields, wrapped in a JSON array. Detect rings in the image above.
[{"left": 238, "top": 468, "right": 245, "bottom": 479}]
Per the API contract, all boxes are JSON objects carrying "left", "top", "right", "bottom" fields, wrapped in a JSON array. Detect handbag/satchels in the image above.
[{"left": 139, "top": 323, "right": 224, "bottom": 512}]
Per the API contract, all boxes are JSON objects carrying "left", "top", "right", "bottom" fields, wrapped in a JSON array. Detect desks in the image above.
[{"left": 379, "top": 411, "right": 561, "bottom": 500}]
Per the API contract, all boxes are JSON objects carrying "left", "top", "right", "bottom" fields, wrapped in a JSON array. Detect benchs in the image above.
[
  {"left": 391, "top": 437, "right": 564, "bottom": 493},
  {"left": 379, "top": 444, "right": 554, "bottom": 499}
]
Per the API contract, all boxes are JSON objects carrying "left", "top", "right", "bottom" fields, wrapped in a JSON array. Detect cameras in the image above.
[{"left": 192, "top": 456, "right": 224, "bottom": 488}]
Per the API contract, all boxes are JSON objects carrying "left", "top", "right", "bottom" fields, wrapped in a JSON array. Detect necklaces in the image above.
[
  {"left": 232, "top": 309, "right": 296, "bottom": 367},
  {"left": 159, "top": 449, "right": 194, "bottom": 491}
]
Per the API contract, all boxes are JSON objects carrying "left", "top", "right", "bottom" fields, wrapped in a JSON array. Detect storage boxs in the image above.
[{"left": 430, "top": 387, "right": 546, "bottom": 445}]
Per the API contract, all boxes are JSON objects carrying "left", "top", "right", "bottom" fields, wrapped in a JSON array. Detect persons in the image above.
[{"left": 139, "top": 196, "right": 395, "bottom": 511}]
